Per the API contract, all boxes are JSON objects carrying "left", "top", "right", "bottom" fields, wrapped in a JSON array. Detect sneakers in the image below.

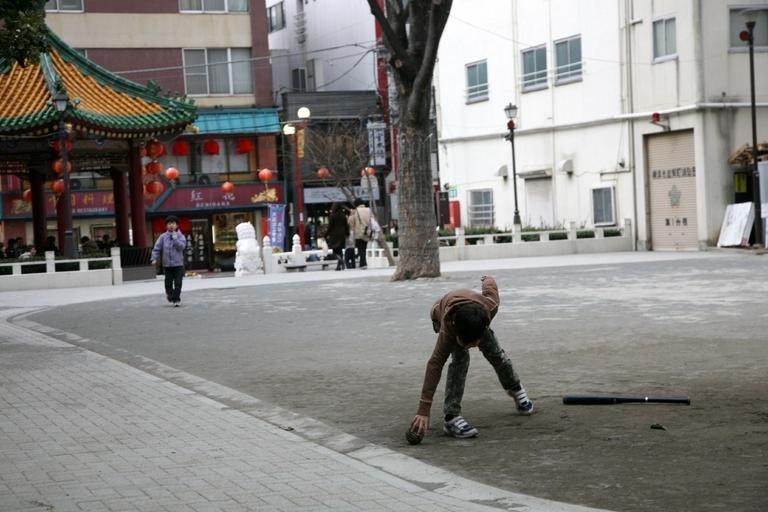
[
  {"left": 443, "top": 415, "right": 478, "bottom": 438},
  {"left": 508, "top": 384, "right": 533, "bottom": 414},
  {"left": 174, "top": 301, "right": 180, "bottom": 306}
]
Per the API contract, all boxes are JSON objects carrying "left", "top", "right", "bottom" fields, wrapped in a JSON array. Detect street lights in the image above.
[
  {"left": 284, "top": 106, "right": 310, "bottom": 250},
  {"left": 506, "top": 104, "right": 523, "bottom": 234},
  {"left": 741, "top": 7, "right": 765, "bottom": 248}
]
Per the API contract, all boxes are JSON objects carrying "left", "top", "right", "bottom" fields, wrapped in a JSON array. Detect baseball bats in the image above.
[{"left": 563, "top": 394, "right": 690, "bottom": 406}]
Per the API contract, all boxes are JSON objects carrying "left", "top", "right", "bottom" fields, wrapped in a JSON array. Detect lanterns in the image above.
[
  {"left": 221, "top": 181, "right": 234, "bottom": 192},
  {"left": 360, "top": 167, "right": 375, "bottom": 177},
  {"left": 258, "top": 167, "right": 272, "bottom": 184},
  {"left": 139, "top": 139, "right": 178, "bottom": 196},
  {"left": 23, "top": 188, "right": 32, "bottom": 202},
  {"left": 53, "top": 140, "right": 72, "bottom": 156},
  {"left": 173, "top": 142, "right": 188, "bottom": 156},
  {"left": 318, "top": 167, "right": 329, "bottom": 178},
  {"left": 52, "top": 158, "right": 72, "bottom": 177},
  {"left": 52, "top": 179, "right": 65, "bottom": 197},
  {"left": 237, "top": 138, "right": 254, "bottom": 155},
  {"left": 204, "top": 141, "right": 219, "bottom": 156}
]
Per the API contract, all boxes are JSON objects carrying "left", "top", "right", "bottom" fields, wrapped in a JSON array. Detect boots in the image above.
[{"left": 334, "top": 254, "right": 345, "bottom": 270}]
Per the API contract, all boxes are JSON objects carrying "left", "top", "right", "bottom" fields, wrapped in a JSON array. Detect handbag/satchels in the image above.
[
  {"left": 361, "top": 225, "right": 376, "bottom": 239},
  {"left": 155, "top": 254, "right": 163, "bottom": 275},
  {"left": 369, "top": 217, "right": 380, "bottom": 239}
]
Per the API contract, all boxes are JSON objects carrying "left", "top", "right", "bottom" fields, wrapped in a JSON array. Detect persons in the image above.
[
  {"left": 409, "top": 275, "right": 533, "bottom": 440},
  {"left": 150, "top": 216, "right": 187, "bottom": 307},
  {"left": 322, "top": 197, "right": 375, "bottom": 272},
  {"left": 0, "top": 234, "right": 120, "bottom": 260}
]
